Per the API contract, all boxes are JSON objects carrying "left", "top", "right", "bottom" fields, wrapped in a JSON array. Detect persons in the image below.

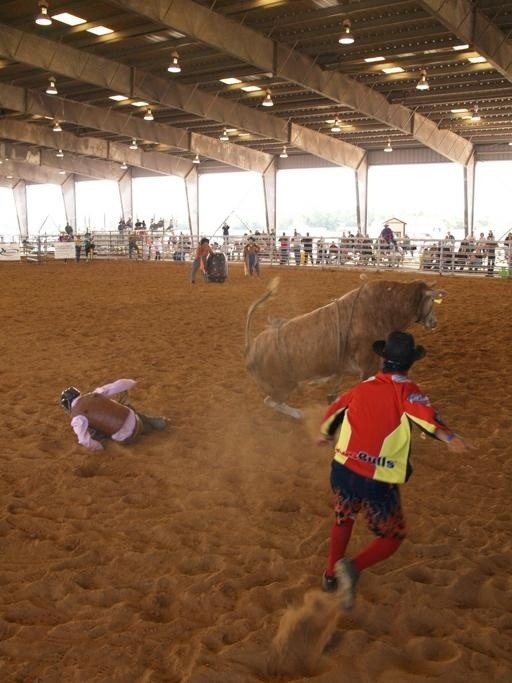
[
  {"left": 60, "top": 378, "right": 169, "bottom": 450},
  {"left": 314, "top": 329, "right": 464, "bottom": 608}
]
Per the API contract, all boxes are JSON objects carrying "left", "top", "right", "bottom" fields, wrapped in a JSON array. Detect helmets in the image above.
[{"left": 60, "top": 385, "right": 81, "bottom": 415}]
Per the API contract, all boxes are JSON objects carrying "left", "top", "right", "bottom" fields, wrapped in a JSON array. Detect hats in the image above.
[{"left": 372, "top": 330, "right": 427, "bottom": 361}]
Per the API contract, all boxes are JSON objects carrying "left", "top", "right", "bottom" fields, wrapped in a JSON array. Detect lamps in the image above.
[
  {"left": 129, "top": 138, "right": 138, "bottom": 149},
  {"left": 383, "top": 137, "right": 393, "bottom": 153},
  {"left": 108, "top": 94, "right": 129, "bottom": 102},
  {"left": 471, "top": 104, "right": 481, "bottom": 121},
  {"left": 45, "top": 72, "right": 58, "bottom": 95},
  {"left": 167, "top": 49, "right": 181, "bottom": 73},
  {"left": 325, "top": 112, "right": 336, "bottom": 123},
  {"left": 56, "top": 149, "right": 64, "bottom": 157},
  {"left": 219, "top": 77, "right": 243, "bottom": 85},
  {"left": 416, "top": 69, "right": 429, "bottom": 89},
  {"left": 453, "top": 44, "right": 468, "bottom": 50},
  {"left": 35, "top": 0, "right": 52, "bottom": 26},
  {"left": 468, "top": 56, "right": 486, "bottom": 63},
  {"left": 262, "top": 88, "right": 274, "bottom": 107},
  {"left": 279, "top": 144, "right": 288, "bottom": 158},
  {"left": 451, "top": 107, "right": 469, "bottom": 113},
  {"left": 86, "top": 25, "right": 114, "bottom": 36},
  {"left": 331, "top": 120, "right": 341, "bottom": 133},
  {"left": 219, "top": 125, "right": 229, "bottom": 141},
  {"left": 192, "top": 154, "right": 200, "bottom": 163},
  {"left": 121, "top": 162, "right": 127, "bottom": 169},
  {"left": 130, "top": 101, "right": 149, "bottom": 107},
  {"left": 51, "top": 12, "right": 86, "bottom": 27},
  {"left": 365, "top": 56, "right": 385, "bottom": 63},
  {"left": 382, "top": 66, "right": 404, "bottom": 74},
  {"left": 143, "top": 104, "right": 154, "bottom": 121},
  {"left": 241, "top": 85, "right": 261, "bottom": 93},
  {"left": 52, "top": 120, "right": 62, "bottom": 132},
  {"left": 338, "top": 18, "right": 354, "bottom": 45}
]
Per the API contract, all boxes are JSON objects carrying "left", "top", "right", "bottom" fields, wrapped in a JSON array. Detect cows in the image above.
[{"left": 245, "top": 277, "right": 449, "bottom": 420}]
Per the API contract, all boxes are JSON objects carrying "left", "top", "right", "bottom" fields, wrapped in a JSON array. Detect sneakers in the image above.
[
  {"left": 322, "top": 570, "right": 337, "bottom": 593},
  {"left": 334, "top": 556, "right": 357, "bottom": 611}
]
